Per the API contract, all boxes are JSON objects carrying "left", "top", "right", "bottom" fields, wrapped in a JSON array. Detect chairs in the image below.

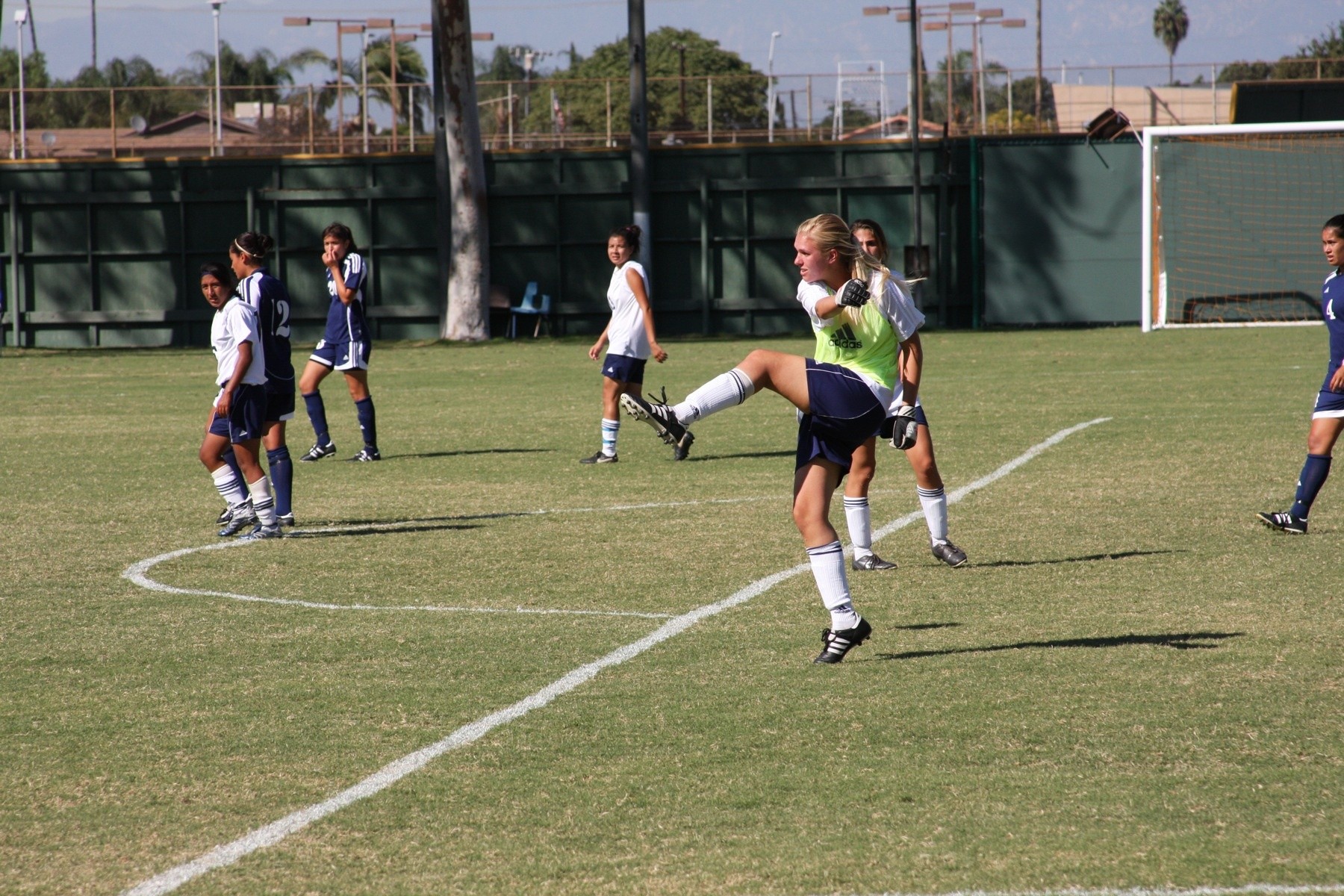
[
  {"left": 489, "top": 283, "right": 513, "bottom": 339},
  {"left": 510, "top": 282, "right": 552, "bottom": 340}
]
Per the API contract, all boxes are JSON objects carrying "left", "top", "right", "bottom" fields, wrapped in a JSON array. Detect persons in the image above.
[
  {"left": 619, "top": 214, "right": 924, "bottom": 664},
  {"left": 216, "top": 233, "right": 302, "bottom": 527},
  {"left": 297, "top": 222, "right": 382, "bottom": 461},
  {"left": 579, "top": 224, "right": 670, "bottom": 464},
  {"left": 1255, "top": 214, "right": 1344, "bottom": 535},
  {"left": 842, "top": 219, "right": 968, "bottom": 571},
  {"left": 198, "top": 261, "right": 284, "bottom": 538}
]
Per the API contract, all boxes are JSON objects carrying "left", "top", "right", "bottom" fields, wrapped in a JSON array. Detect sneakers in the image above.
[
  {"left": 239, "top": 521, "right": 283, "bottom": 540},
  {"left": 355, "top": 448, "right": 381, "bottom": 461},
  {"left": 621, "top": 386, "right": 690, "bottom": 445},
  {"left": 217, "top": 493, "right": 253, "bottom": 525},
  {"left": 275, "top": 512, "right": 295, "bottom": 527},
  {"left": 931, "top": 539, "right": 969, "bottom": 568},
  {"left": 852, "top": 551, "right": 897, "bottom": 570},
  {"left": 814, "top": 611, "right": 872, "bottom": 663},
  {"left": 673, "top": 432, "right": 696, "bottom": 460},
  {"left": 581, "top": 451, "right": 618, "bottom": 463},
  {"left": 218, "top": 501, "right": 260, "bottom": 536},
  {"left": 1257, "top": 509, "right": 1308, "bottom": 534},
  {"left": 300, "top": 440, "right": 336, "bottom": 462}
]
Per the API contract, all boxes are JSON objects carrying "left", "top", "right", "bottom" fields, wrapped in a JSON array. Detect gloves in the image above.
[
  {"left": 889, "top": 405, "right": 918, "bottom": 449},
  {"left": 835, "top": 279, "right": 872, "bottom": 309}
]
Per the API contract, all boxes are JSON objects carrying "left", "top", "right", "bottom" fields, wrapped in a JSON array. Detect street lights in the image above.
[
  {"left": 207, "top": 1, "right": 230, "bottom": 158},
  {"left": 13, "top": 6, "right": 30, "bottom": 162},
  {"left": 765, "top": 31, "right": 788, "bottom": 145},
  {"left": 862, "top": 1, "right": 976, "bottom": 139},
  {"left": 340, "top": 22, "right": 433, "bottom": 154},
  {"left": 925, "top": 17, "right": 1028, "bottom": 137},
  {"left": 282, "top": 17, "right": 395, "bottom": 155},
  {"left": 897, "top": 7, "right": 1005, "bottom": 137}
]
